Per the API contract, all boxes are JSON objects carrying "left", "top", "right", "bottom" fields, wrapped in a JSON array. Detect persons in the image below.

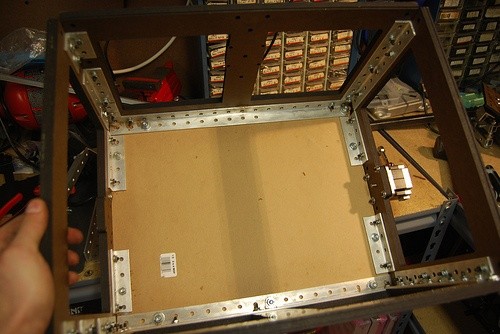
[{"left": 1, "top": 197, "right": 84, "bottom": 334}]
